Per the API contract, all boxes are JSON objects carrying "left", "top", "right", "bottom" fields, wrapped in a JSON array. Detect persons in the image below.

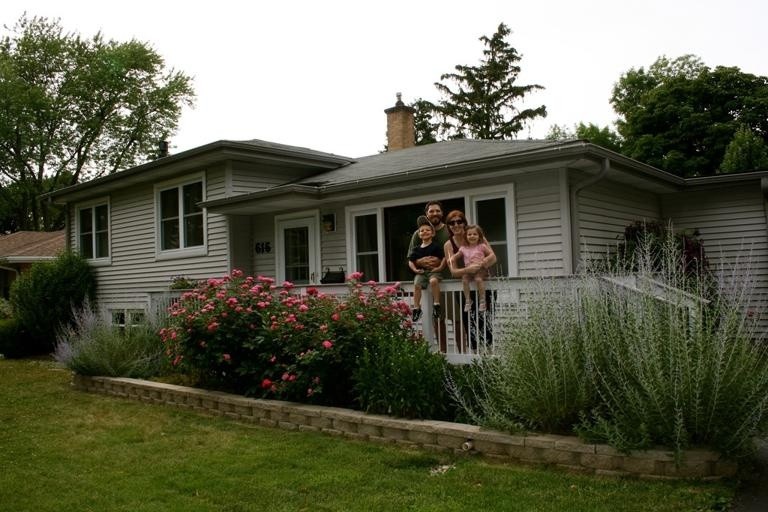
[
  {"left": 442, "top": 210, "right": 497, "bottom": 357},
  {"left": 407, "top": 201, "right": 469, "bottom": 355},
  {"left": 407, "top": 215, "right": 445, "bottom": 323},
  {"left": 447, "top": 222, "right": 497, "bottom": 313}
]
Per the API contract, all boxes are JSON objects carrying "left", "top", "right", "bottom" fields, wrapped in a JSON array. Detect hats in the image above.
[{"left": 417, "top": 215, "right": 434, "bottom": 230}]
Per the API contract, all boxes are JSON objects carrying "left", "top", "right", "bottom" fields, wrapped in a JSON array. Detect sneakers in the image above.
[
  {"left": 479, "top": 302, "right": 486, "bottom": 312},
  {"left": 464, "top": 300, "right": 474, "bottom": 312},
  {"left": 412, "top": 308, "right": 423, "bottom": 322},
  {"left": 433, "top": 303, "right": 442, "bottom": 320}
]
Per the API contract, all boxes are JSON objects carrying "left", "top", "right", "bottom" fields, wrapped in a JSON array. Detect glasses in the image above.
[{"left": 449, "top": 219, "right": 463, "bottom": 226}]
